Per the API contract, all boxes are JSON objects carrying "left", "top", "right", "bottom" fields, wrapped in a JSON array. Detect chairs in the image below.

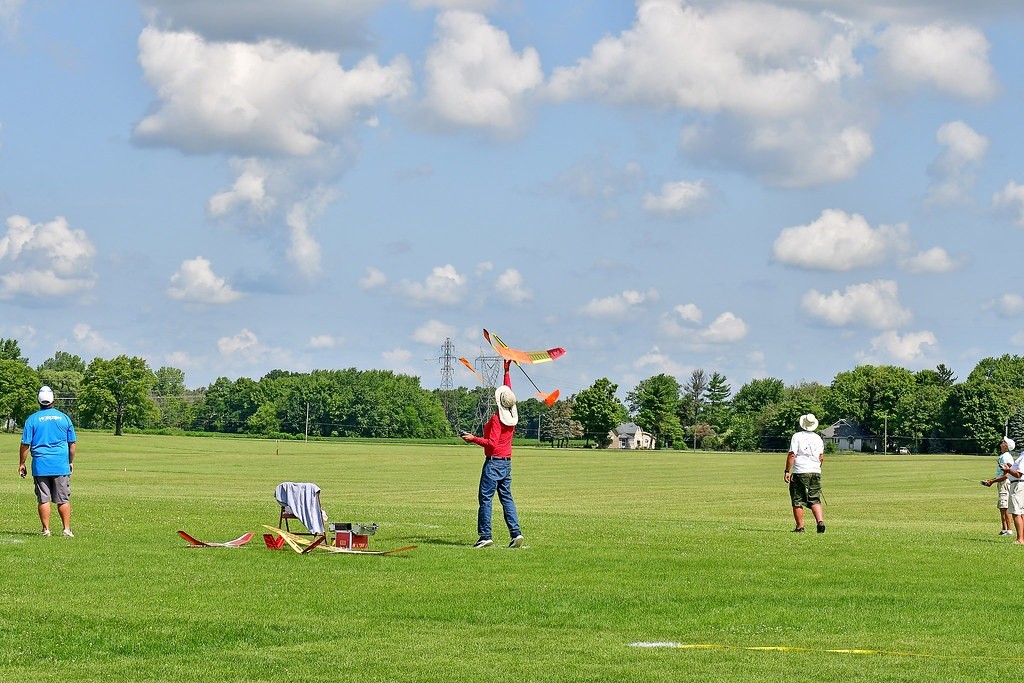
[{"left": 274, "top": 482, "right": 328, "bottom": 545}]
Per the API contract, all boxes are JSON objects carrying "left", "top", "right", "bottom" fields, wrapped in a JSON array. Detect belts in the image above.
[
  {"left": 1010, "top": 479, "right": 1024, "bottom": 483},
  {"left": 486, "top": 456, "right": 511, "bottom": 461}
]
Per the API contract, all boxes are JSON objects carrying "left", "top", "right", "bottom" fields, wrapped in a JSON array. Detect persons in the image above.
[
  {"left": 462, "top": 359, "right": 524, "bottom": 548},
  {"left": 987, "top": 436, "right": 1015, "bottom": 535},
  {"left": 784, "top": 414, "right": 826, "bottom": 533},
  {"left": 19, "top": 386, "right": 76, "bottom": 537},
  {"left": 1001, "top": 451, "right": 1024, "bottom": 543}
]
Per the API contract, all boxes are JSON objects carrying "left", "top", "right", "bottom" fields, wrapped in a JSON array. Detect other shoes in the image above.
[
  {"left": 41, "top": 530, "right": 51, "bottom": 537},
  {"left": 62, "top": 529, "right": 74, "bottom": 537},
  {"left": 999, "top": 530, "right": 1007, "bottom": 535},
  {"left": 1011, "top": 539, "right": 1024, "bottom": 545},
  {"left": 1001, "top": 530, "right": 1013, "bottom": 536}
]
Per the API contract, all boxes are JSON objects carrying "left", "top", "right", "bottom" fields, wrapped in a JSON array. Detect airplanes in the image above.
[
  {"left": 482, "top": 328, "right": 567, "bottom": 407},
  {"left": 260, "top": 523, "right": 417, "bottom": 555},
  {"left": 177, "top": 530, "right": 255, "bottom": 550}
]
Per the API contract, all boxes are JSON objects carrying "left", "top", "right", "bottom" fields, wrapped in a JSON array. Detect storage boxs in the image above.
[{"left": 336, "top": 531, "right": 368, "bottom": 549}]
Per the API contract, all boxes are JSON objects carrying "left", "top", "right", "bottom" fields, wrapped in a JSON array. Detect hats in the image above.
[
  {"left": 799, "top": 414, "right": 819, "bottom": 431},
  {"left": 38, "top": 386, "right": 54, "bottom": 405},
  {"left": 494, "top": 385, "right": 518, "bottom": 425},
  {"left": 1004, "top": 436, "right": 1015, "bottom": 451}
]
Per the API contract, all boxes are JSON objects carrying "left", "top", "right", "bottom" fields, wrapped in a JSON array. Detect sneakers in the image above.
[
  {"left": 817, "top": 521, "right": 825, "bottom": 533},
  {"left": 473, "top": 538, "right": 494, "bottom": 548},
  {"left": 508, "top": 534, "right": 524, "bottom": 548},
  {"left": 790, "top": 526, "right": 805, "bottom": 533}
]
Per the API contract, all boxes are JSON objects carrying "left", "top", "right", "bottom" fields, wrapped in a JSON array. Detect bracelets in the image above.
[{"left": 785, "top": 470, "right": 789, "bottom": 473}]
